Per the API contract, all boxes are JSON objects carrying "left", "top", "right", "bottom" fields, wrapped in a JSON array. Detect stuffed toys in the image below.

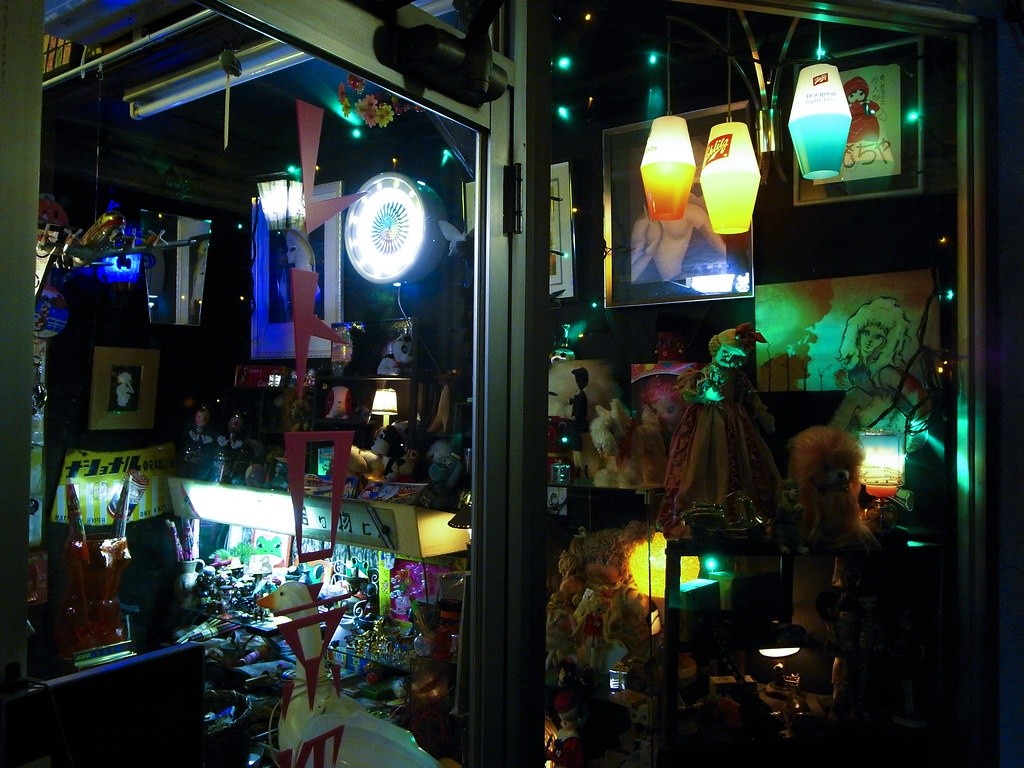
[
  {"left": 544, "top": 312, "right": 918, "bottom": 768},
  {"left": 287, "top": 338, "right": 466, "bottom": 492}
]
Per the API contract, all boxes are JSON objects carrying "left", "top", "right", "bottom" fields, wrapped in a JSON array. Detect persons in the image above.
[
  {"left": 545, "top": 691, "right": 588, "bottom": 768},
  {"left": 436, "top": 600, "right": 461, "bottom": 634},
  {"left": 657, "top": 323, "right": 788, "bottom": 527},
  {"left": 643, "top": 379, "right": 688, "bottom": 448}
]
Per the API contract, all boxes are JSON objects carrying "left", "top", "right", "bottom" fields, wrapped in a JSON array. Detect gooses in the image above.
[{"left": 255, "top": 579, "right": 443, "bottom": 768}]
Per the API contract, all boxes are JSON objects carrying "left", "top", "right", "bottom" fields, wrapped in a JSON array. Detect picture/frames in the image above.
[
  {"left": 793, "top": 41, "right": 925, "bottom": 207},
  {"left": 603, "top": 100, "right": 754, "bottom": 308},
  {"left": 87, "top": 345, "right": 160, "bottom": 431}
]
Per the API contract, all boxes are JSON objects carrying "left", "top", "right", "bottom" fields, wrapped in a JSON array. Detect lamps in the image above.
[
  {"left": 639, "top": 19, "right": 696, "bottom": 221},
  {"left": 787, "top": 22, "right": 852, "bottom": 180},
  {"left": 713, "top": 623, "right": 806, "bottom": 728},
  {"left": 371, "top": 388, "right": 398, "bottom": 427},
  {"left": 316, "top": 161, "right": 447, "bottom": 286},
  {"left": 699, "top": 9, "right": 761, "bottom": 235},
  {"left": 122, "top": 0, "right": 455, "bottom": 122}
]
